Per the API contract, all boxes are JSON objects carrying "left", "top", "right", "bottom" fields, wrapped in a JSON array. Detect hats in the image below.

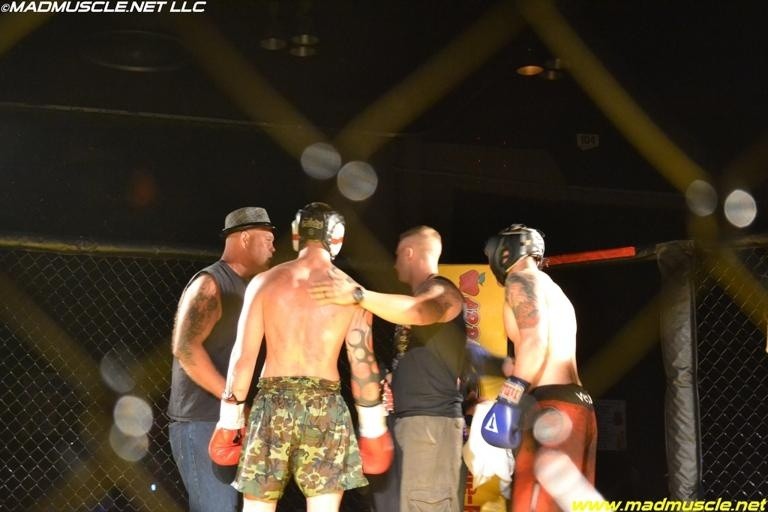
[{"left": 218, "top": 206, "right": 280, "bottom": 240}]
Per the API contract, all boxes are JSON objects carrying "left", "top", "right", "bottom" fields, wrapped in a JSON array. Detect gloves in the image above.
[
  {"left": 461, "top": 339, "right": 508, "bottom": 384},
  {"left": 354, "top": 402, "right": 395, "bottom": 476},
  {"left": 208, "top": 400, "right": 246, "bottom": 466},
  {"left": 481, "top": 375, "right": 530, "bottom": 450}
]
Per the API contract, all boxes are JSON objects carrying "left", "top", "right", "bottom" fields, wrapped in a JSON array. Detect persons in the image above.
[
  {"left": 167, "top": 203, "right": 277, "bottom": 512},
  {"left": 460, "top": 336, "right": 517, "bottom": 447},
  {"left": 206, "top": 201, "right": 399, "bottom": 510},
  {"left": 306, "top": 222, "right": 469, "bottom": 512},
  {"left": 483, "top": 221, "right": 615, "bottom": 512}
]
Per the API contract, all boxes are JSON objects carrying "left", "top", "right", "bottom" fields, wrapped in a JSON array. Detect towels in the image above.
[{"left": 458, "top": 400, "right": 513, "bottom": 500}]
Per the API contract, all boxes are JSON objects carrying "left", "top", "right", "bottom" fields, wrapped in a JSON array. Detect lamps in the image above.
[
  {"left": 258, "top": 23, "right": 328, "bottom": 59},
  {"left": 510, "top": 37, "right": 579, "bottom": 82}
]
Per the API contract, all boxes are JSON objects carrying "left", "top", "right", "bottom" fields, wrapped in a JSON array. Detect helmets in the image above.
[
  {"left": 484, "top": 224, "right": 545, "bottom": 287},
  {"left": 290, "top": 202, "right": 347, "bottom": 261}
]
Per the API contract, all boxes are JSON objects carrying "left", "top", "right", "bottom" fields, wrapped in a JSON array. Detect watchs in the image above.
[{"left": 351, "top": 286, "right": 365, "bottom": 307}]
